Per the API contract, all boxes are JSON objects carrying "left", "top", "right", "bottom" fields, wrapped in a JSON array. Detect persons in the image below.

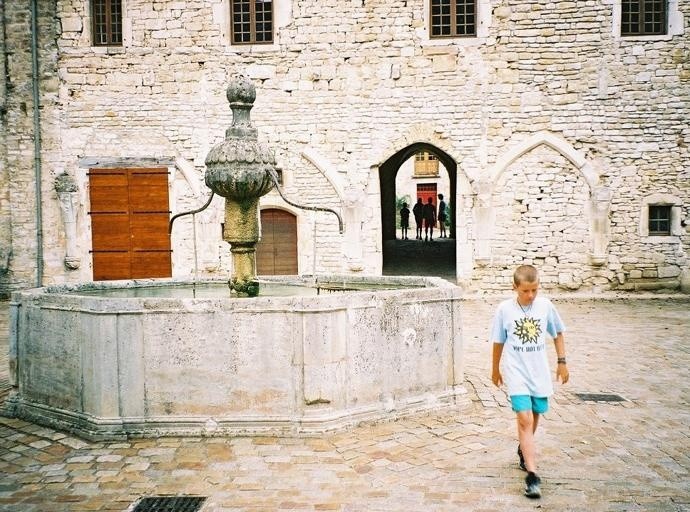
[
  {"left": 401, "top": 194, "right": 448, "bottom": 241},
  {"left": 491, "top": 266, "right": 569, "bottom": 497}
]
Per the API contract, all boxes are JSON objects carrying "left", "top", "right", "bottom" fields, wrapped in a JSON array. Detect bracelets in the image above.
[{"left": 557, "top": 358, "right": 566, "bottom": 364}]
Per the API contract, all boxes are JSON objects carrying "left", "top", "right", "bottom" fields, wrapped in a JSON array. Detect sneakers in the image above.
[
  {"left": 516, "top": 444, "right": 527, "bottom": 472},
  {"left": 523, "top": 470, "right": 545, "bottom": 499},
  {"left": 401, "top": 234, "right": 447, "bottom": 243}
]
